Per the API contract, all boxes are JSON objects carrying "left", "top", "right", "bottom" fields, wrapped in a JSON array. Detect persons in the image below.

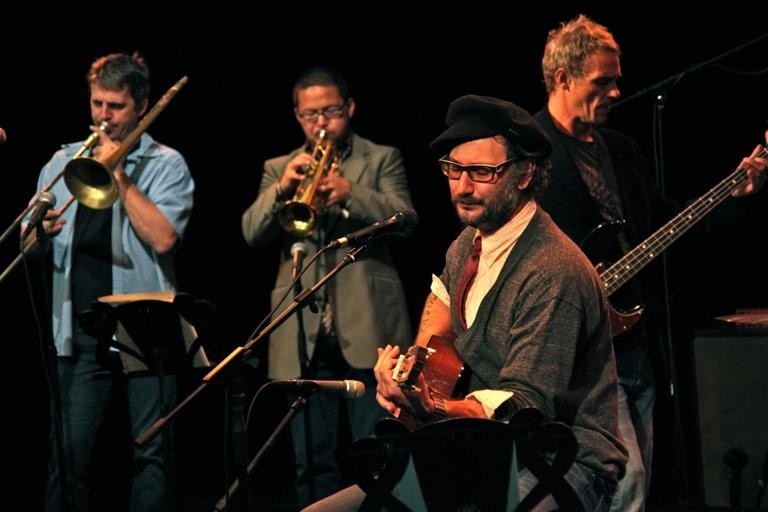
[
  {"left": 240, "top": 68, "right": 419, "bottom": 511},
  {"left": 19, "top": 53, "right": 199, "bottom": 511},
  {"left": 532, "top": 13, "right": 767, "bottom": 511},
  {"left": 302, "top": 94, "right": 634, "bottom": 512}
]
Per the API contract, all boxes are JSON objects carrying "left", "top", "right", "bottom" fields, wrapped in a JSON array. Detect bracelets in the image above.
[{"left": 432, "top": 396, "right": 449, "bottom": 420}]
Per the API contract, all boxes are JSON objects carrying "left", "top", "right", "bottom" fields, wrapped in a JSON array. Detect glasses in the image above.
[
  {"left": 297, "top": 99, "right": 346, "bottom": 120},
  {"left": 439, "top": 152, "right": 522, "bottom": 182}
]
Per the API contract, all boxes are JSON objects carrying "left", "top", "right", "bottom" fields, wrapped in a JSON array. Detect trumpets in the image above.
[{"left": 274, "top": 128, "right": 339, "bottom": 239}]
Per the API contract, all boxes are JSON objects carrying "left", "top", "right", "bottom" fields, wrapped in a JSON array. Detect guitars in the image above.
[
  {"left": 590, "top": 139, "right": 768, "bottom": 343},
  {"left": 388, "top": 327, "right": 467, "bottom": 433}
]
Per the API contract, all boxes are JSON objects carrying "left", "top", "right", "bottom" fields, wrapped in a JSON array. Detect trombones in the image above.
[{"left": 0, "top": 74, "right": 190, "bottom": 283}]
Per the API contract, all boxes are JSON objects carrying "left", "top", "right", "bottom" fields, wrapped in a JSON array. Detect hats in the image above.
[{"left": 429, "top": 94, "right": 554, "bottom": 153}]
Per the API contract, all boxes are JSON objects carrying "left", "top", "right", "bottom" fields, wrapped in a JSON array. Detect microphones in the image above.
[
  {"left": 290, "top": 242, "right": 308, "bottom": 282},
  {"left": 331, "top": 207, "right": 418, "bottom": 248},
  {"left": 20, "top": 192, "right": 56, "bottom": 240},
  {"left": 269, "top": 378, "right": 365, "bottom": 400}
]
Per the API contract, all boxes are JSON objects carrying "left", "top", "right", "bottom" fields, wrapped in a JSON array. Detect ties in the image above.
[{"left": 456, "top": 238, "right": 481, "bottom": 329}]
